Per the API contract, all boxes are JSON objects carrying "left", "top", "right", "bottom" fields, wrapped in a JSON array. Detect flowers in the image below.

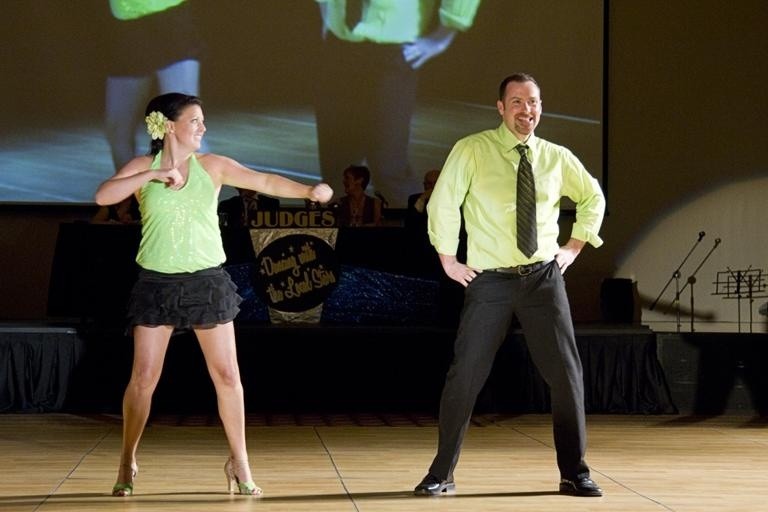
[{"left": 145, "top": 111, "right": 168, "bottom": 141}]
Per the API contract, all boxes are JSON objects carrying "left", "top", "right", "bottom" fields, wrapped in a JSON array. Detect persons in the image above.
[
  {"left": 314, "top": 0, "right": 482, "bottom": 207},
  {"left": 103, "top": 0, "right": 201, "bottom": 172},
  {"left": 218, "top": 188, "right": 281, "bottom": 229},
  {"left": 91, "top": 193, "right": 141, "bottom": 224},
  {"left": 414, "top": 72, "right": 606, "bottom": 496},
  {"left": 406, "top": 170, "right": 440, "bottom": 223},
  {"left": 328, "top": 165, "right": 384, "bottom": 226},
  {"left": 93, "top": 91, "right": 334, "bottom": 497}
]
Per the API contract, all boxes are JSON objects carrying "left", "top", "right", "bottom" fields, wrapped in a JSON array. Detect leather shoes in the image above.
[
  {"left": 559, "top": 479, "right": 602, "bottom": 497},
  {"left": 413, "top": 473, "right": 455, "bottom": 496}
]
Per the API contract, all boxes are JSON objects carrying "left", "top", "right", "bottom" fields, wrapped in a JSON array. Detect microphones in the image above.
[
  {"left": 698, "top": 231, "right": 705, "bottom": 242},
  {"left": 374, "top": 190, "right": 390, "bottom": 206},
  {"left": 715, "top": 237, "right": 721, "bottom": 248}
]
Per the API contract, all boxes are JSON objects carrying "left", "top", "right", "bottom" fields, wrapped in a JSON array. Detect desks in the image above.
[{"left": 50, "top": 224, "right": 466, "bottom": 325}]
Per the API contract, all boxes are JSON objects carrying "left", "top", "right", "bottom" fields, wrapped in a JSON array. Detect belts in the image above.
[{"left": 487, "top": 261, "right": 543, "bottom": 275}]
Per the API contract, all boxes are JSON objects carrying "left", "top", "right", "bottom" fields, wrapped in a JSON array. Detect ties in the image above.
[
  {"left": 343, "top": 0, "right": 363, "bottom": 32},
  {"left": 515, "top": 144, "right": 537, "bottom": 259}
]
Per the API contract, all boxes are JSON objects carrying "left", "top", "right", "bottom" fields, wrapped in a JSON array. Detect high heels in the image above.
[
  {"left": 113, "top": 462, "right": 138, "bottom": 497},
  {"left": 224, "top": 457, "right": 263, "bottom": 497}
]
